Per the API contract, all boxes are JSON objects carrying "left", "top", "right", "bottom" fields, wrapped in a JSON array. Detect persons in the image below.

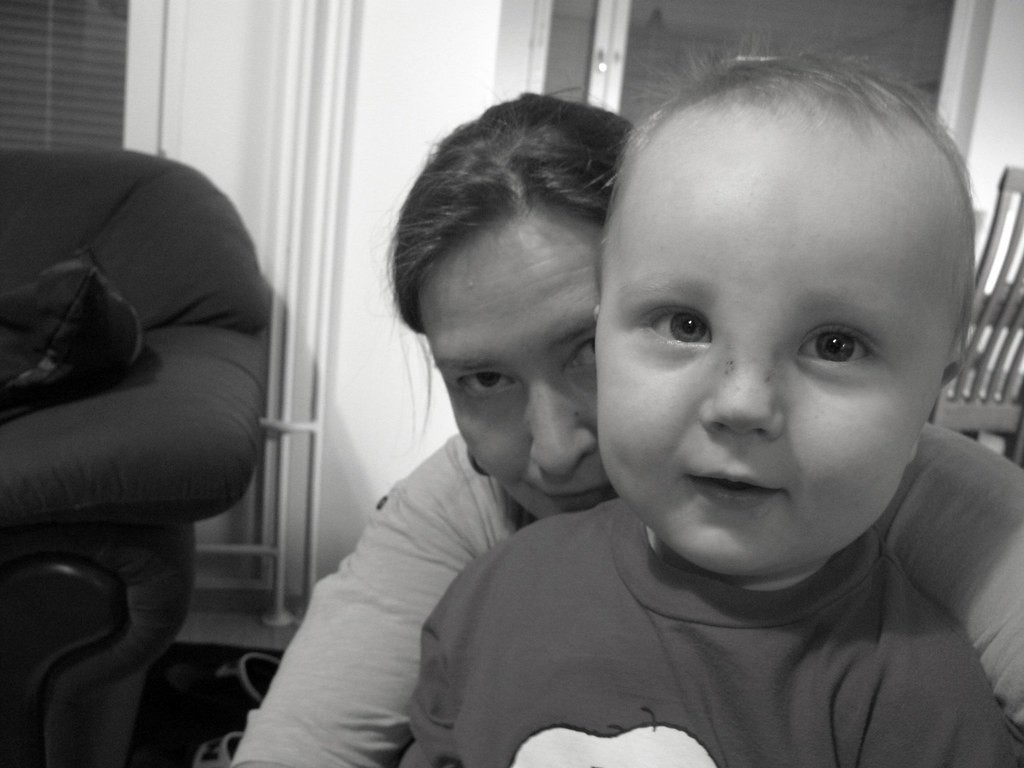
[
  {"left": 229, "top": 90, "right": 1024, "bottom": 768},
  {"left": 400, "top": 50, "right": 1023, "bottom": 767}
]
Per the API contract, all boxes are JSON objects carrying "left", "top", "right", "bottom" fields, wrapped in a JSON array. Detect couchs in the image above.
[{"left": 0, "top": 141, "right": 274, "bottom": 768}]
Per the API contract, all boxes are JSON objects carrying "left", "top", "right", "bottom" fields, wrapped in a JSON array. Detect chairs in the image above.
[{"left": 923, "top": 164, "right": 1024, "bottom": 456}]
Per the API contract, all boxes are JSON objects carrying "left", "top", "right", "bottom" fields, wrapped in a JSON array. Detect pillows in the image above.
[{"left": 0, "top": 239, "right": 144, "bottom": 423}]
